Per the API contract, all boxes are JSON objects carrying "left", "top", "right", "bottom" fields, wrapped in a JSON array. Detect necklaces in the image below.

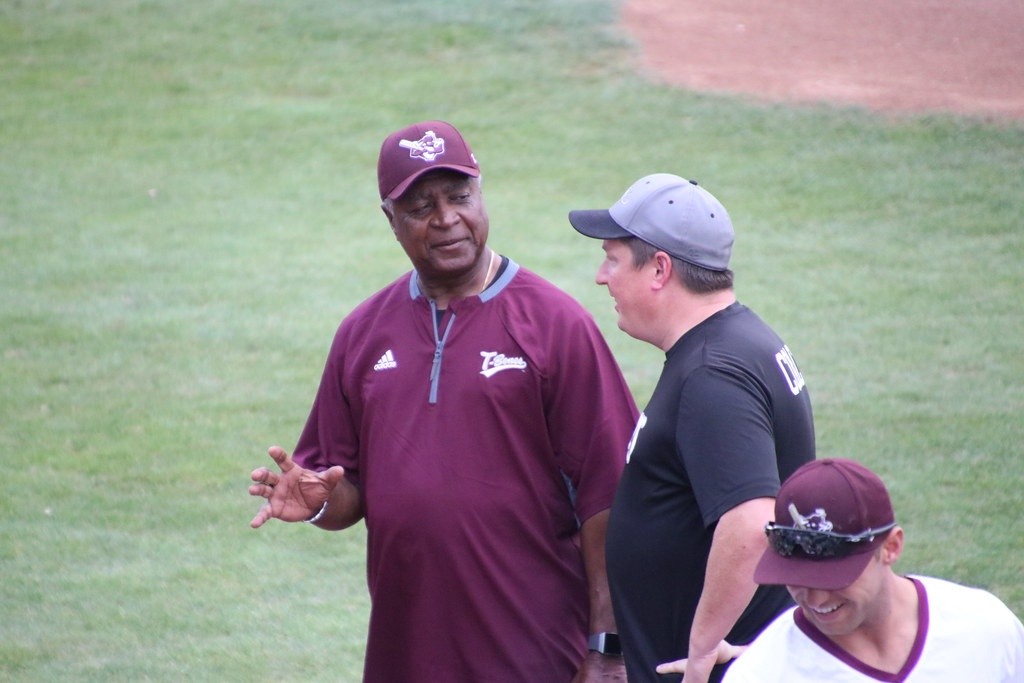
[{"left": 481, "top": 250, "right": 494, "bottom": 292}]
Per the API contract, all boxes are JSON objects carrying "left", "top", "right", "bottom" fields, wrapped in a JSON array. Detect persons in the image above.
[
  {"left": 718, "top": 458, "right": 1024, "bottom": 683},
  {"left": 569, "top": 171, "right": 815, "bottom": 683},
  {"left": 247, "top": 120, "right": 641, "bottom": 683}
]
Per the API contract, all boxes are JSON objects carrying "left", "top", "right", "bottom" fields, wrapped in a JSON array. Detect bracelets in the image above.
[{"left": 302, "top": 501, "right": 327, "bottom": 524}]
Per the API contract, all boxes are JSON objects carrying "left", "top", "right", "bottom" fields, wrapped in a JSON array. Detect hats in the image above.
[
  {"left": 568, "top": 174, "right": 735, "bottom": 272},
  {"left": 378, "top": 121, "right": 480, "bottom": 201},
  {"left": 754, "top": 458, "right": 894, "bottom": 591}
]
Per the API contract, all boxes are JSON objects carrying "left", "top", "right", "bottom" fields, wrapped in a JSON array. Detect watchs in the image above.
[{"left": 587, "top": 633, "right": 623, "bottom": 657}]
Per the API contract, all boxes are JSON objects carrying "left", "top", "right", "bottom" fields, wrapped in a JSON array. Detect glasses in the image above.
[{"left": 765, "top": 521, "right": 897, "bottom": 560}]
